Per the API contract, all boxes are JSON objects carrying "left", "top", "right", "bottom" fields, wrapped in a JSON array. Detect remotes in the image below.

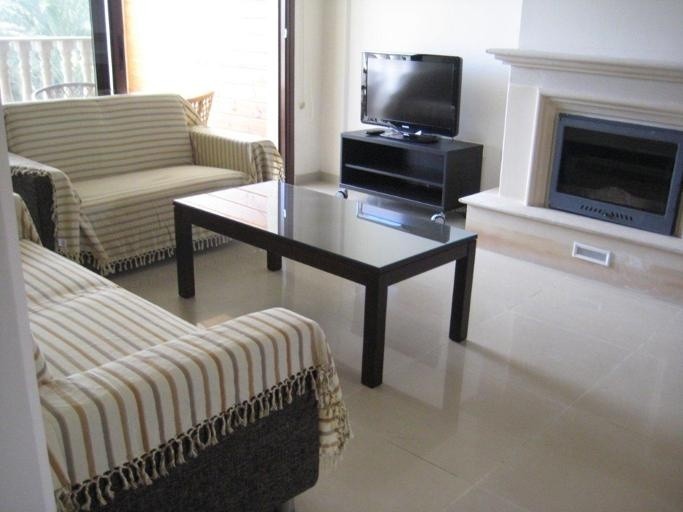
[{"left": 366, "top": 130, "right": 385, "bottom": 135}]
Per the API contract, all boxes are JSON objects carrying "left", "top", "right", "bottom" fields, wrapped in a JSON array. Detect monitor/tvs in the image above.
[{"left": 361, "top": 51, "right": 462, "bottom": 143}]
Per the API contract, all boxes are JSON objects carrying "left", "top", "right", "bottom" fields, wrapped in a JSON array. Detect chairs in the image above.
[
  {"left": 187, "top": 91, "right": 218, "bottom": 122},
  {"left": 31, "top": 83, "right": 96, "bottom": 98}
]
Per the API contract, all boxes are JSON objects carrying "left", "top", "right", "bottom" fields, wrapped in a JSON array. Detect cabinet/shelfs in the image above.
[{"left": 339, "top": 129, "right": 484, "bottom": 212}]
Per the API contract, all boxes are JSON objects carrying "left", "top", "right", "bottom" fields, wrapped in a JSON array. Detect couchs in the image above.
[
  {"left": 12, "top": 185, "right": 350, "bottom": 507},
  {"left": 2, "top": 92, "right": 277, "bottom": 275}
]
